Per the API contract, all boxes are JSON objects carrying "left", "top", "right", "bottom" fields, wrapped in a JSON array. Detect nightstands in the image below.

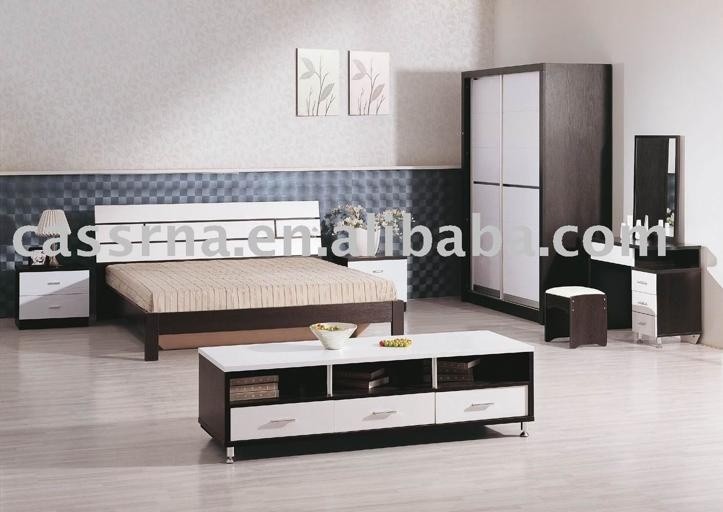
[
  {"left": 320, "top": 253, "right": 408, "bottom": 313},
  {"left": 14, "top": 262, "right": 97, "bottom": 330}
]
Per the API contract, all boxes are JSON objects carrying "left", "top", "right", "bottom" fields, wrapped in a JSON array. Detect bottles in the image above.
[{"left": 619, "top": 214, "right": 670, "bottom": 244}]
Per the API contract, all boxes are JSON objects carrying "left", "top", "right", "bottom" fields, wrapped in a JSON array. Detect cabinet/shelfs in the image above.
[{"left": 459, "top": 61, "right": 613, "bottom": 326}]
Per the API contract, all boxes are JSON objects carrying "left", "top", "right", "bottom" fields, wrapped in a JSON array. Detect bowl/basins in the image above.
[{"left": 309, "top": 322, "right": 358, "bottom": 350}]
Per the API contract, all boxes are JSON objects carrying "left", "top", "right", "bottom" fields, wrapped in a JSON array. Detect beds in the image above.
[{"left": 93, "top": 202, "right": 405, "bottom": 362}]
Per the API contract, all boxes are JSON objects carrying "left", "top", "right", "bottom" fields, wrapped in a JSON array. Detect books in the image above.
[
  {"left": 422, "top": 356, "right": 481, "bottom": 383},
  {"left": 333, "top": 364, "right": 390, "bottom": 391},
  {"left": 229, "top": 375, "right": 279, "bottom": 402}
]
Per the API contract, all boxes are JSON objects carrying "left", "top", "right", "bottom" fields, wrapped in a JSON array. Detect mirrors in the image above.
[{"left": 632, "top": 135, "right": 681, "bottom": 246}]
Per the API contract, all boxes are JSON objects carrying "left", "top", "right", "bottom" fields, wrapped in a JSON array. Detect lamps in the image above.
[{"left": 34, "top": 209, "right": 72, "bottom": 266}]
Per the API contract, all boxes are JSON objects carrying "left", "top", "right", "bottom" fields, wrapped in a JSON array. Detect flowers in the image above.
[
  {"left": 324, "top": 201, "right": 381, "bottom": 235},
  {"left": 374, "top": 206, "right": 416, "bottom": 239}
]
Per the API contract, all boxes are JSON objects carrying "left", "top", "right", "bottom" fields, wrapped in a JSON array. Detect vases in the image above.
[
  {"left": 348, "top": 226, "right": 379, "bottom": 256},
  {"left": 384, "top": 226, "right": 393, "bottom": 257}
]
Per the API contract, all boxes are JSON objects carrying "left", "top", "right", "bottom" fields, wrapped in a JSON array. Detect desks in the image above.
[
  {"left": 573, "top": 236, "right": 703, "bottom": 350},
  {"left": 194, "top": 329, "right": 536, "bottom": 465}
]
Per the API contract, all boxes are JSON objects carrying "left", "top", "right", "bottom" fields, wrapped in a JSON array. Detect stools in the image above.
[{"left": 542, "top": 285, "right": 610, "bottom": 350}]
